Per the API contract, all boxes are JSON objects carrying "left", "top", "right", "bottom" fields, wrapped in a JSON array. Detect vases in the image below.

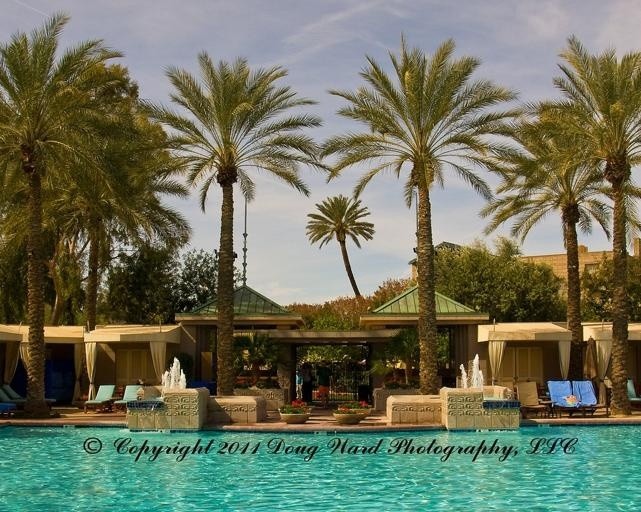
[{"left": 280, "top": 414, "right": 366, "bottom": 424}]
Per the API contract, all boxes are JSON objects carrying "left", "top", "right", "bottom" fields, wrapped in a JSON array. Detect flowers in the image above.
[{"left": 277, "top": 400, "right": 371, "bottom": 414}]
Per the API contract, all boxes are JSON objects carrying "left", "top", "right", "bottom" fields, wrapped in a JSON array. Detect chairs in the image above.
[
  {"left": 492, "top": 376, "right": 641, "bottom": 418},
  {"left": 84, "top": 384, "right": 142, "bottom": 415},
  {"left": 0, "top": 384, "right": 57, "bottom": 418}
]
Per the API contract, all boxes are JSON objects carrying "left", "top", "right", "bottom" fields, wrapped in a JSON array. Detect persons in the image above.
[
  {"left": 302, "top": 362, "right": 315, "bottom": 403},
  {"left": 316, "top": 359, "right": 334, "bottom": 410}
]
